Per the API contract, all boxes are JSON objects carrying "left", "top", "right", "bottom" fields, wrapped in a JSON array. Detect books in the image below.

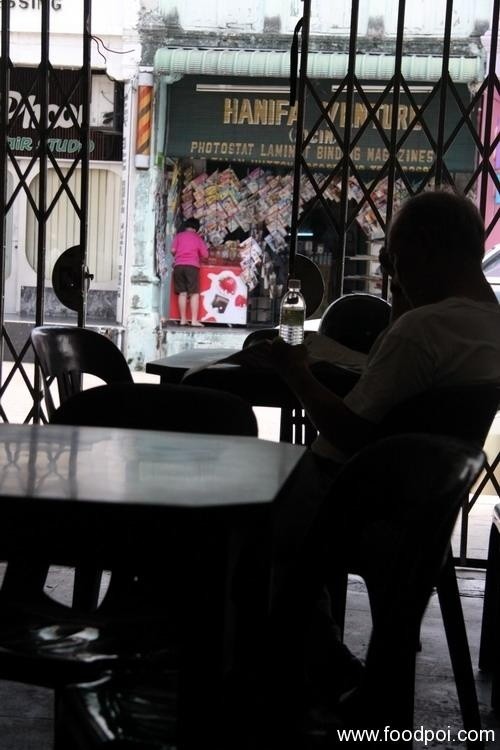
[{"left": 177, "top": 330, "right": 373, "bottom": 385}]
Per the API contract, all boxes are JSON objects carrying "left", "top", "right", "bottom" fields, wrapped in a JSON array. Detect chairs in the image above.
[
  {"left": 331, "top": 444, "right": 492, "bottom": 741},
  {"left": 243, "top": 326, "right": 320, "bottom": 442},
  {"left": 22, "top": 319, "right": 136, "bottom": 426},
  {"left": 322, "top": 289, "right": 399, "bottom": 343},
  {"left": 1, "top": 379, "right": 257, "bottom": 749},
  {"left": 260, "top": 382, "right": 500, "bottom": 750}
]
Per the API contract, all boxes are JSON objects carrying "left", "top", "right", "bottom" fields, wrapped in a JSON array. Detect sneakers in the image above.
[{"left": 180, "top": 319, "right": 205, "bottom": 327}]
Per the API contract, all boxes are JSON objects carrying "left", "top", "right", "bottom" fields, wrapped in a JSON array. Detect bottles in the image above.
[{"left": 278, "top": 278, "right": 306, "bottom": 348}]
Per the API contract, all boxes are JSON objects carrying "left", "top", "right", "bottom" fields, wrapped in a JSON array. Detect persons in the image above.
[
  {"left": 217, "top": 188, "right": 500, "bottom": 749},
  {"left": 170, "top": 217, "right": 211, "bottom": 329}
]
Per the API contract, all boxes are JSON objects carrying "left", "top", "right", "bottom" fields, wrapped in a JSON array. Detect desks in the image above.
[
  {"left": 146, "top": 341, "right": 317, "bottom": 446},
  {"left": 1, "top": 421, "right": 312, "bottom": 748}
]
